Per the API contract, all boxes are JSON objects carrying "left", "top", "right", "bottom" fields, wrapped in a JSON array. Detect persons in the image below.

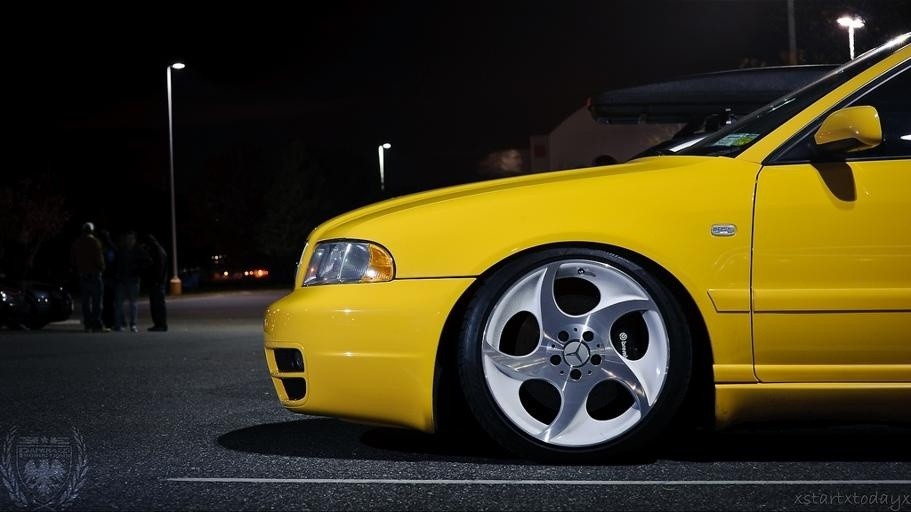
[
  {"left": 625, "top": 126, "right": 665, "bottom": 157},
  {"left": 78, "top": 222, "right": 112, "bottom": 332},
  {"left": 2, "top": 230, "right": 34, "bottom": 331},
  {"left": 96, "top": 229, "right": 128, "bottom": 327},
  {"left": 110, "top": 229, "right": 144, "bottom": 333},
  {"left": 471, "top": 143, "right": 526, "bottom": 180},
  {"left": 140, "top": 235, "right": 172, "bottom": 332}
]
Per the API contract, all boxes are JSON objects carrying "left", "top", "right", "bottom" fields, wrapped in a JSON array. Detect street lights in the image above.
[
  {"left": 166, "top": 62, "right": 186, "bottom": 296},
  {"left": 378, "top": 141, "right": 392, "bottom": 190},
  {"left": 836, "top": 14, "right": 866, "bottom": 61}
]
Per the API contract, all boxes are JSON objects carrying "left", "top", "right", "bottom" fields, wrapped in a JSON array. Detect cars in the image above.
[
  {"left": 264, "top": 31, "right": 911, "bottom": 464},
  {"left": 0, "top": 259, "right": 72, "bottom": 330}
]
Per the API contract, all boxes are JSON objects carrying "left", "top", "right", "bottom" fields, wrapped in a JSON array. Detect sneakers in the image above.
[
  {"left": 85, "top": 325, "right": 138, "bottom": 334},
  {"left": 147, "top": 324, "right": 168, "bottom": 332}
]
